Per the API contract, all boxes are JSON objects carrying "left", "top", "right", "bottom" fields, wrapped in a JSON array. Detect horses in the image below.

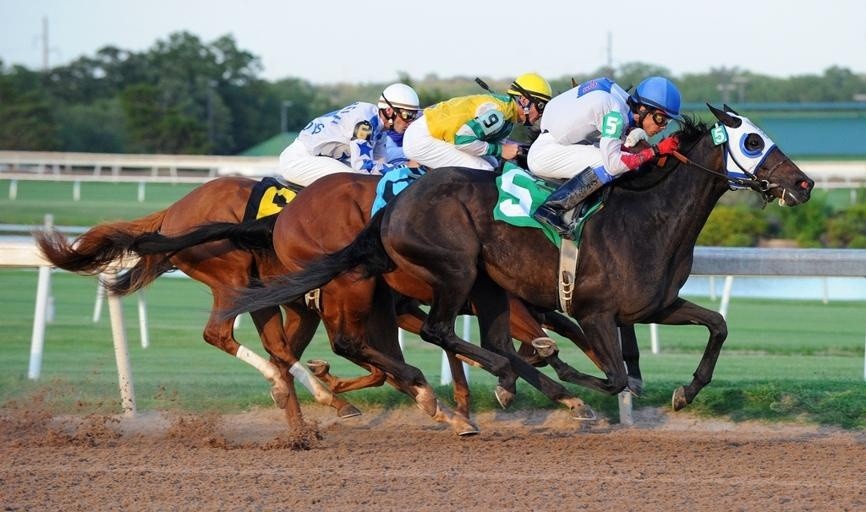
[{"left": 29, "top": 76, "right": 815, "bottom": 442}]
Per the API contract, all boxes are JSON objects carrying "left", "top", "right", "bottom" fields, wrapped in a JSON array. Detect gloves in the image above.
[{"left": 654, "top": 136, "right": 679, "bottom": 157}]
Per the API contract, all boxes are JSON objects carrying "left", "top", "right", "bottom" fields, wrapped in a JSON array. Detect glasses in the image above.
[
  {"left": 534, "top": 98, "right": 547, "bottom": 114},
  {"left": 648, "top": 108, "right": 668, "bottom": 127},
  {"left": 398, "top": 110, "right": 417, "bottom": 121}
]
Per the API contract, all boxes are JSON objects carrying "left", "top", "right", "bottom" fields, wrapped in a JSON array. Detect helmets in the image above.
[
  {"left": 377, "top": 83, "right": 425, "bottom": 111},
  {"left": 507, "top": 72, "right": 552, "bottom": 101},
  {"left": 632, "top": 76, "right": 684, "bottom": 120}
]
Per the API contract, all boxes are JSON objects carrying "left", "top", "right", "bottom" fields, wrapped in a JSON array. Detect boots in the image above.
[{"left": 532, "top": 166, "right": 604, "bottom": 240}]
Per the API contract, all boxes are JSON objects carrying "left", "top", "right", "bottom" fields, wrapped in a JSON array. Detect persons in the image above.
[
  {"left": 277, "top": 82, "right": 420, "bottom": 188},
  {"left": 373, "top": 109, "right": 424, "bottom": 165},
  {"left": 526, "top": 75, "right": 686, "bottom": 243},
  {"left": 403, "top": 70, "right": 552, "bottom": 179}
]
